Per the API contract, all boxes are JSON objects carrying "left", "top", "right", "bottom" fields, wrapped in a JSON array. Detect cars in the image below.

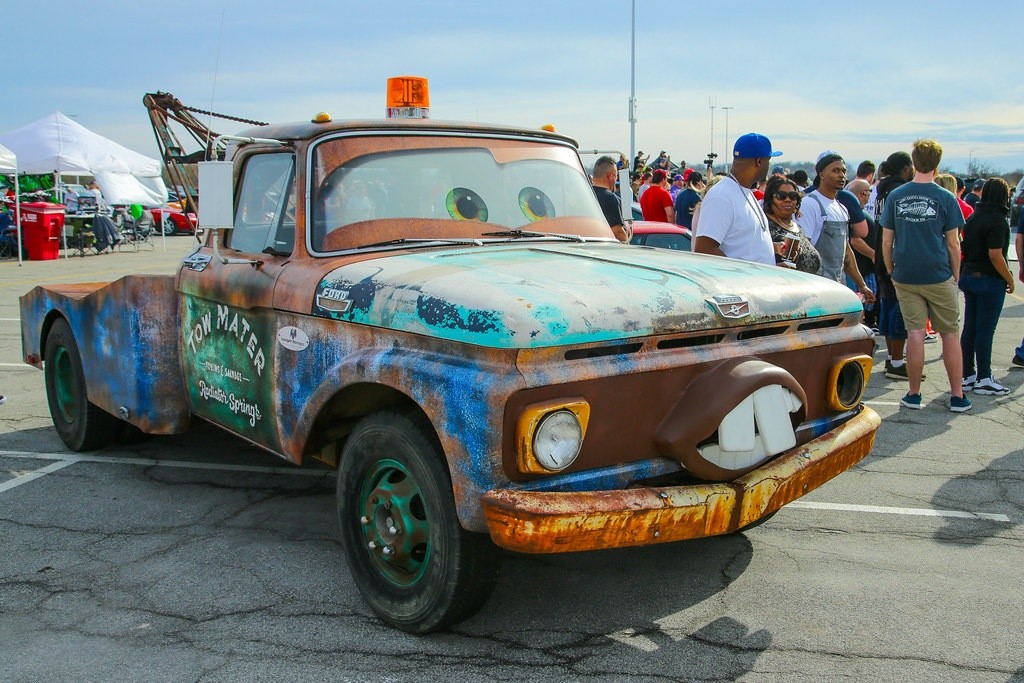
[
  {"left": 631, "top": 203, "right": 643, "bottom": 221},
  {"left": 149, "top": 208, "right": 199, "bottom": 235},
  {"left": 166, "top": 190, "right": 198, "bottom": 210},
  {"left": 624, "top": 221, "right": 692, "bottom": 255}
]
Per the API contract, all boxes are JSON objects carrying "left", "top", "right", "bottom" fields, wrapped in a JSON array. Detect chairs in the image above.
[
  {"left": 59, "top": 210, "right": 154, "bottom": 256},
  {"left": 0, "top": 212, "right": 17, "bottom": 259}
]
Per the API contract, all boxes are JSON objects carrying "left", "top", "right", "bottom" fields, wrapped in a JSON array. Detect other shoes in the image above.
[
  {"left": 1012, "top": 354, "right": 1024, "bottom": 368},
  {"left": 869, "top": 325, "right": 881, "bottom": 335},
  {"left": 923, "top": 332, "right": 939, "bottom": 343},
  {"left": 928, "top": 329, "right": 940, "bottom": 338}
]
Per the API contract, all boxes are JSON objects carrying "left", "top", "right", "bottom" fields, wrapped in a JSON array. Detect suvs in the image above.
[
  {"left": 1010, "top": 175, "right": 1024, "bottom": 226},
  {"left": 963, "top": 178, "right": 977, "bottom": 199}
]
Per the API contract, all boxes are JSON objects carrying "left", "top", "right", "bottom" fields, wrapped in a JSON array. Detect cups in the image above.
[{"left": 781, "top": 232, "right": 800, "bottom": 261}]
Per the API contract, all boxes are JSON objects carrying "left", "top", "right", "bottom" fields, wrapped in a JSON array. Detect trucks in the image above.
[{"left": 0, "top": 166, "right": 115, "bottom": 218}]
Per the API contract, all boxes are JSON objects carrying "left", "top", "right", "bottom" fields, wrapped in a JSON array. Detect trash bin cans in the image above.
[{"left": 11, "top": 202, "right": 66, "bottom": 260}]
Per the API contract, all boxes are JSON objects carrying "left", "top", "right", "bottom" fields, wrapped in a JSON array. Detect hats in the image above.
[
  {"left": 674, "top": 175, "right": 683, "bottom": 181},
  {"left": 973, "top": 179, "right": 986, "bottom": 189},
  {"left": 733, "top": 133, "right": 783, "bottom": 159},
  {"left": 654, "top": 170, "right": 667, "bottom": 179},
  {"left": 684, "top": 169, "right": 694, "bottom": 181},
  {"left": 772, "top": 166, "right": 784, "bottom": 174}
]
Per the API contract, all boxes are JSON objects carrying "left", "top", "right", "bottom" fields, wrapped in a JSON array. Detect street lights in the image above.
[{"left": 721, "top": 107, "right": 733, "bottom": 165}]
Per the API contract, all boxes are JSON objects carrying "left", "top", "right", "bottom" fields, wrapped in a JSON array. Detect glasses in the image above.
[{"left": 773, "top": 191, "right": 800, "bottom": 201}]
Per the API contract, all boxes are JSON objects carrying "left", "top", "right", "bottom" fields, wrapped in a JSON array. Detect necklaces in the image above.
[
  {"left": 728, "top": 172, "right": 766, "bottom": 231},
  {"left": 775, "top": 215, "right": 794, "bottom": 230}
]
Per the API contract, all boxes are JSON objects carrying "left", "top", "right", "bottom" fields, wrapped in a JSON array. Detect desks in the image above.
[{"left": 62, "top": 213, "right": 95, "bottom": 257}]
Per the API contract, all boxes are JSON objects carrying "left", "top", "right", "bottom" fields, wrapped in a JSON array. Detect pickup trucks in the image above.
[{"left": 19, "top": 76, "right": 881, "bottom": 635}]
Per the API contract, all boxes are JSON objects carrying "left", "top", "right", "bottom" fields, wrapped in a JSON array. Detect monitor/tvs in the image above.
[{"left": 77, "top": 196, "right": 96, "bottom": 212}]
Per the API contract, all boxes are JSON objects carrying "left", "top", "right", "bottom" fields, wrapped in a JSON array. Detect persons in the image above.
[
  {"left": 959, "top": 177, "right": 1014, "bottom": 396},
  {"left": 591, "top": 156, "right": 630, "bottom": 245},
  {"left": 64, "top": 182, "right": 102, "bottom": 213},
  {"left": 879, "top": 138, "right": 974, "bottom": 412},
  {"left": 275, "top": 133, "right": 1024, "bottom": 382}
]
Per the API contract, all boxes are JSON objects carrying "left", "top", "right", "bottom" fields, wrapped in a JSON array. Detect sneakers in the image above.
[
  {"left": 883, "top": 359, "right": 926, "bottom": 380},
  {"left": 901, "top": 390, "right": 921, "bottom": 409},
  {"left": 973, "top": 375, "right": 1010, "bottom": 396},
  {"left": 962, "top": 370, "right": 977, "bottom": 392},
  {"left": 949, "top": 393, "right": 972, "bottom": 412}
]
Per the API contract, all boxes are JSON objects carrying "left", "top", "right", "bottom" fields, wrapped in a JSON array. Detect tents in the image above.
[
  {"left": 0, "top": 145, "right": 26, "bottom": 265},
  {"left": 0, "top": 111, "right": 169, "bottom": 258}
]
